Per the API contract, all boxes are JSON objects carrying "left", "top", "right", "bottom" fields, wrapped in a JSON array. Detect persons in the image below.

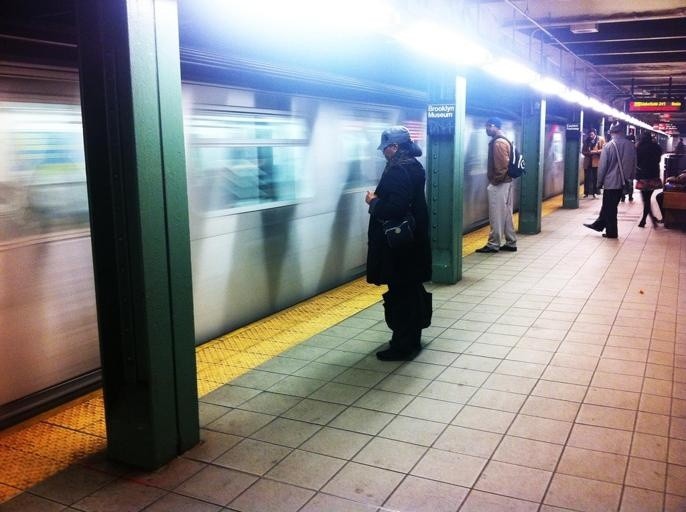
[
  {"left": 475, "top": 117, "right": 517, "bottom": 252},
  {"left": 582, "top": 121, "right": 685, "bottom": 238},
  {"left": 365, "top": 126, "right": 432, "bottom": 360}
]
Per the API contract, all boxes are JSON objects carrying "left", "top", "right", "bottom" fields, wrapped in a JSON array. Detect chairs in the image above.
[{"left": 663, "top": 191, "right": 686, "bottom": 228}]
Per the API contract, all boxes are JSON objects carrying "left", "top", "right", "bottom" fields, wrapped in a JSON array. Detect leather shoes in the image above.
[
  {"left": 475, "top": 246, "right": 497, "bottom": 252},
  {"left": 602, "top": 233, "right": 617, "bottom": 238},
  {"left": 499, "top": 245, "right": 517, "bottom": 251},
  {"left": 376, "top": 348, "right": 407, "bottom": 361},
  {"left": 583, "top": 223, "right": 603, "bottom": 231}
]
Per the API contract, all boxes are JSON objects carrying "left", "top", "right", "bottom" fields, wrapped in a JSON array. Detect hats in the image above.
[
  {"left": 607, "top": 121, "right": 622, "bottom": 135},
  {"left": 377, "top": 126, "right": 410, "bottom": 150},
  {"left": 487, "top": 117, "right": 502, "bottom": 128}
]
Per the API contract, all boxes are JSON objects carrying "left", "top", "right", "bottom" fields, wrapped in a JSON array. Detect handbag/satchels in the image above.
[
  {"left": 382, "top": 290, "right": 432, "bottom": 330},
  {"left": 379, "top": 210, "right": 417, "bottom": 249},
  {"left": 621, "top": 179, "right": 633, "bottom": 195}
]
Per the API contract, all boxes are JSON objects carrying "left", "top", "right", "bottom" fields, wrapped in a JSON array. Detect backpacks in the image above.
[{"left": 492, "top": 135, "right": 528, "bottom": 178}]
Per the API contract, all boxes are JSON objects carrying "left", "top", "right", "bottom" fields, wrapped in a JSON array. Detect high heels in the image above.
[
  {"left": 652, "top": 216, "right": 660, "bottom": 224},
  {"left": 638, "top": 220, "right": 645, "bottom": 227}
]
